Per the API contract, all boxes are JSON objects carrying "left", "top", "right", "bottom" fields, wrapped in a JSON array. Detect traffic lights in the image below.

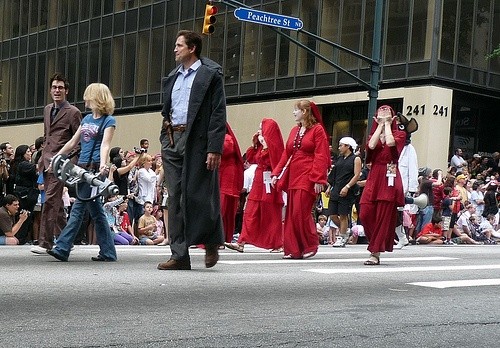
[{"left": 202, "top": 4, "right": 218, "bottom": 36}]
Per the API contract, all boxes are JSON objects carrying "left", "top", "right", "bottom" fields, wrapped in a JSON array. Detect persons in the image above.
[
  {"left": 223, "top": 119, "right": 286, "bottom": 254},
  {"left": 469, "top": 151, "right": 500, "bottom": 178},
  {"left": 313, "top": 147, "right": 372, "bottom": 244},
  {"left": 402, "top": 164, "right": 500, "bottom": 245},
  {"left": 31, "top": 72, "right": 83, "bottom": 254},
  {"left": 156, "top": 30, "right": 227, "bottom": 269},
  {"left": 391, "top": 113, "right": 419, "bottom": 249},
  {"left": 269, "top": 100, "right": 332, "bottom": 261},
  {"left": 451, "top": 147, "right": 467, "bottom": 174},
  {"left": 357, "top": 106, "right": 407, "bottom": 265},
  {"left": 100, "top": 138, "right": 171, "bottom": 246},
  {"left": 0, "top": 137, "right": 48, "bottom": 245},
  {"left": 234, "top": 151, "right": 258, "bottom": 244},
  {"left": 61, "top": 185, "right": 98, "bottom": 246},
  {"left": 189, "top": 121, "right": 244, "bottom": 250},
  {"left": 45, "top": 83, "right": 118, "bottom": 261},
  {"left": 324, "top": 137, "right": 363, "bottom": 249}
]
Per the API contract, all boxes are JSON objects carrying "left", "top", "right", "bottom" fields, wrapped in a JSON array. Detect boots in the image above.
[{"left": 393, "top": 225, "right": 409, "bottom": 249}]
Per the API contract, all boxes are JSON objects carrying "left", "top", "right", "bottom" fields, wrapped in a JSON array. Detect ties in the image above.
[{"left": 50, "top": 108, "right": 57, "bottom": 124}]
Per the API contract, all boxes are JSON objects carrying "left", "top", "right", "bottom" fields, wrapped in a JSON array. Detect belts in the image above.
[{"left": 172, "top": 125, "right": 186, "bottom": 130}]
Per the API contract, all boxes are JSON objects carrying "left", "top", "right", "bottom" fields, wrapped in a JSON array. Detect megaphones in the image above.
[{"left": 405, "top": 193, "right": 429, "bottom": 209}]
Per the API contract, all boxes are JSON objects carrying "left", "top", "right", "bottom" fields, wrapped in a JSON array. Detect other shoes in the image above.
[
  {"left": 205, "top": 249, "right": 218, "bottom": 268},
  {"left": 30, "top": 245, "right": 48, "bottom": 254},
  {"left": 443, "top": 239, "right": 499, "bottom": 246},
  {"left": 46, "top": 249, "right": 68, "bottom": 261},
  {"left": 157, "top": 259, "right": 191, "bottom": 270},
  {"left": 333, "top": 237, "right": 346, "bottom": 247},
  {"left": 343, "top": 228, "right": 353, "bottom": 243},
  {"left": 92, "top": 255, "right": 114, "bottom": 262}
]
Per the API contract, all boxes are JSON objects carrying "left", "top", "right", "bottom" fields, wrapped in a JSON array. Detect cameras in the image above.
[
  {"left": 135, "top": 148, "right": 147, "bottom": 154},
  {"left": 21, "top": 209, "right": 32, "bottom": 216}
]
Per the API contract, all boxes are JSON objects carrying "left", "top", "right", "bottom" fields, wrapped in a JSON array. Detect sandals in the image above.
[
  {"left": 270, "top": 247, "right": 284, "bottom": 253},
  {"left": 224, "top": 242, "right": 244, "bottom": 253},
  {"left": 364, "top": 255, "right": 380, "bottom": 265}
]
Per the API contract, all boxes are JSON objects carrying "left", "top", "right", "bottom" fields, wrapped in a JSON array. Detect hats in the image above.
[
  {"left": 457, "top": 175, "right": 468, "bottom": 180},
  {"left": 423, "top": 168, "right": 431, "bottom": 176}
]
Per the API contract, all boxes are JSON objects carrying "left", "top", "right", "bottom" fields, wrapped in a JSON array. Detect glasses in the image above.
[{"left": 51, "top": 86, "right": 66, "bottom": 90}]
